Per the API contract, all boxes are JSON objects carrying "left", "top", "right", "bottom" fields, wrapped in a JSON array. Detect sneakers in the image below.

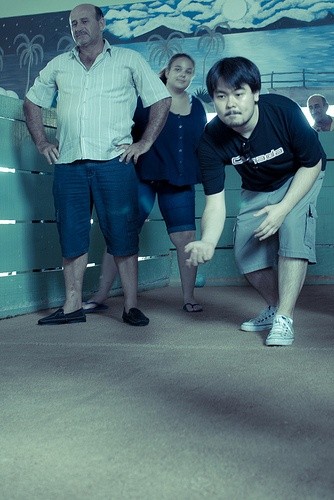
[
  {"left": 241, "top": 306, "right": 277, "bottom": 331},
  {"left": 266, "top": 315, "right": 295, "bottom": 346}
]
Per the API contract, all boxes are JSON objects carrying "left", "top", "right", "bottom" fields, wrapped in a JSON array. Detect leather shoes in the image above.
[
  {"left": 38, "top": 307, "right": 86, "bottom": 325},
  {"left": 122, "top": 306, "right": 149, "bottom": 327}
]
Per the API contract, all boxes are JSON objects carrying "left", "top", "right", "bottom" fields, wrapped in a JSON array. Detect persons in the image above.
[
  {"left": 183, "top": 55, "right": 326, "bottom": 346},
  {"left": 79, "top": 51, "right": 209, "bottom": 313},
  {"left": 307, "top": 93, "right": 333, "bottom": 132},
  {"left": 23, "top": 3, "right": 174, "bottom": 327}
]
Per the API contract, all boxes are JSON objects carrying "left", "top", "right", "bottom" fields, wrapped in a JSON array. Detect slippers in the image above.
[
  {"left": 184, "top": 303, "right": 203, "bottom": 312},
  {"left": 83, "top": 299, "right": 109, "bottom": 313}
]
